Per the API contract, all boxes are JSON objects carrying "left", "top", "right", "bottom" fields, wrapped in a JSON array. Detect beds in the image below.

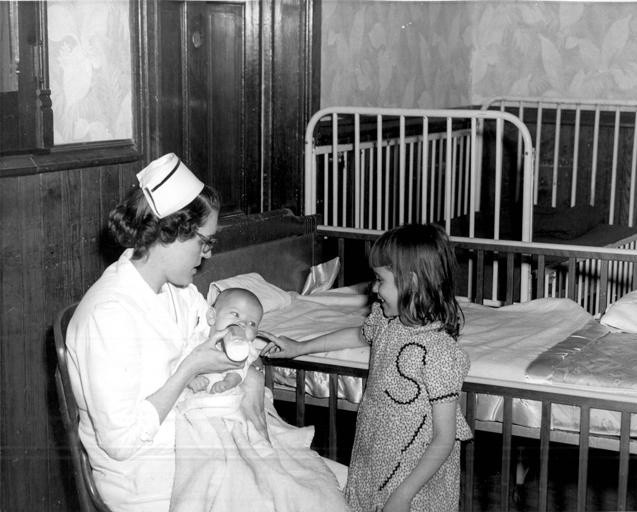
[
  {"left": 303, "top": 98, "right": 636, "bottom": 305},
  {"left": 204, "top": 278, "right": 636, "bottom": 511}
]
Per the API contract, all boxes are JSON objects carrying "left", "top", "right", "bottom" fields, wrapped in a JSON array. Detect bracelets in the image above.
[{"left": 248, "top": 363, "right": 266, "bottom": 376}]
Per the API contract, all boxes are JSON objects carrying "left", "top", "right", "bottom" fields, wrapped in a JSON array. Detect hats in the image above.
[{"left": 136, "top": 151, "right": 205, "bottom": 219}]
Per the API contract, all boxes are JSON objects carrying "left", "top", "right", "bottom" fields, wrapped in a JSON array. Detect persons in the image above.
[
  {"left": 66, "top": 151, "right": 351, "bottom": 512},
  {"left": 255, "top": 224, "right": 475, "bottom": 512},
  {"left": 179, "top": 287, "right": 264, "bottom": 396}
]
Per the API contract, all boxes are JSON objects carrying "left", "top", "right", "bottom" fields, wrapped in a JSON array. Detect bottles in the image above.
[{"left": 223, "top": 324, "right": 250, "bottom": 361}]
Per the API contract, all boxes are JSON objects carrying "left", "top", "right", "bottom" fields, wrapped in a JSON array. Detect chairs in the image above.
[{"left": 50, "top": 301, "right": 111, "bottom": 512}]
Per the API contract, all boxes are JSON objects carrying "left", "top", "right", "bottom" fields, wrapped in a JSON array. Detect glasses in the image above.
[{"left": 191, "top": 231, "right": 218, "bottom": 254}]
